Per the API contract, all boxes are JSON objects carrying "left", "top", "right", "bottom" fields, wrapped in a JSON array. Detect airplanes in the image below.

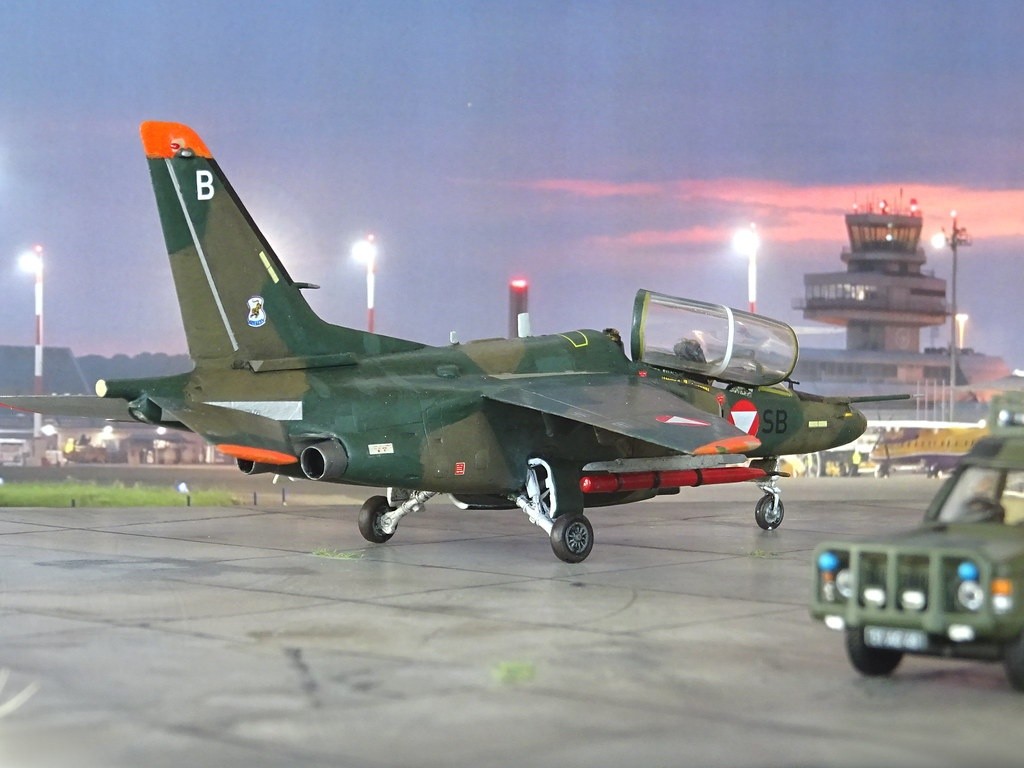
[{"left": 2, "top": 118, "right": 915, "bottom": 563}]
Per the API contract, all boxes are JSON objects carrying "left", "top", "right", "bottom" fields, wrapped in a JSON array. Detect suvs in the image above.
[{"left": 804, "top": 391, "right": 1023, "bottom": 690}]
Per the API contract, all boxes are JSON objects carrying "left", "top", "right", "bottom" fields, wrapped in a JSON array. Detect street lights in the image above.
[
  {"left": 13, "top": 245, "right": 46, "bottom": 440},
  {"left": 930, "top": 209, "right": 971, "bottom": 421},
  {"left": 729, "top": 222, "right": 758, "bottom": 314},
  {"left": 351, "top": 234, "right": 375, "bottom": 331}
]
